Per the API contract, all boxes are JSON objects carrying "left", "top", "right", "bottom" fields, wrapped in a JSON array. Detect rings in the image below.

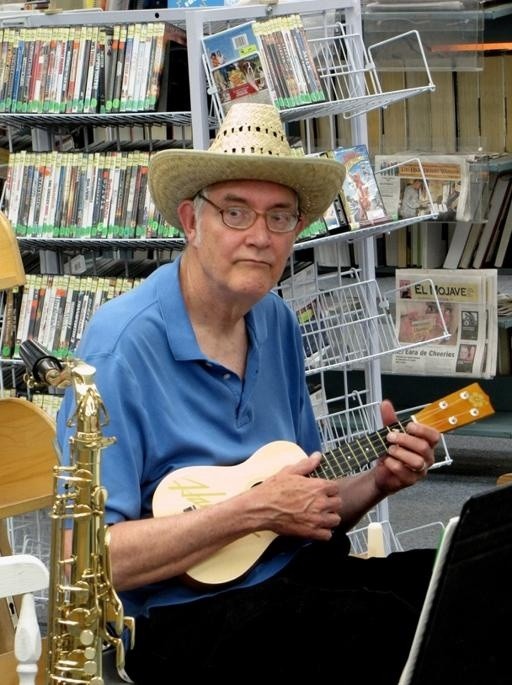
[{"left": 409, "top": 461, "right": 427, "bottom": 473}]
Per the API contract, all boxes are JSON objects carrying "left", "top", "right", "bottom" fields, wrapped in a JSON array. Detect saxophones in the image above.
[{"left": 19, "top": 339, "right": 136, "bottom": 685}]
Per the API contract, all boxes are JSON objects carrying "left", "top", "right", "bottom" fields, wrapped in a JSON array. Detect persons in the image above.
[{"left": 49, "top": 101, "right": 443, "bottom": 685}]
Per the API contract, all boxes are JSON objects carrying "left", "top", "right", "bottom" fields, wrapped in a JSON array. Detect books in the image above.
[
  {"left": 202, "top": 13, "right": 326, "bottom": 117},
  {"left": 276, "top": 259, "right": 332, "bottom": 356},
  {"left": 288, "top": 136, "right": 390, "bottom": 242},
  {"left": 0, "top": 124, "right": 194, "bottom": 238},
  {"left": 0, "top": 0, "right": 166, "bottom": 17},
  {"left": 316, "top": 49, "right": 512, "bottom": 155},
  {"left": 1, "top": 21, "right": 191, "bottom": 114},
  {"left": 1, "top": 247, "right": 180, "bottom": 360},
  {"left": 312, "top": 172, "right": 511, "bottom": 273}
]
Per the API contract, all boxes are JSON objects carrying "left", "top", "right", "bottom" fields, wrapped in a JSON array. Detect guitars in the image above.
[{"left": 152, "top": 382, "right": 495, "bottom": 585}]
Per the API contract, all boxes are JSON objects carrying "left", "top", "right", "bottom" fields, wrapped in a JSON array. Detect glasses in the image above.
[{"left": 198, "top": 192, "right": 301, "bottom": 233}]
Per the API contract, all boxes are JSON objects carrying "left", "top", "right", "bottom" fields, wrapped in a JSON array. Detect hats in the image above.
[{"left": 147, "top": 100, "right": 348, "bottom": 239}]
